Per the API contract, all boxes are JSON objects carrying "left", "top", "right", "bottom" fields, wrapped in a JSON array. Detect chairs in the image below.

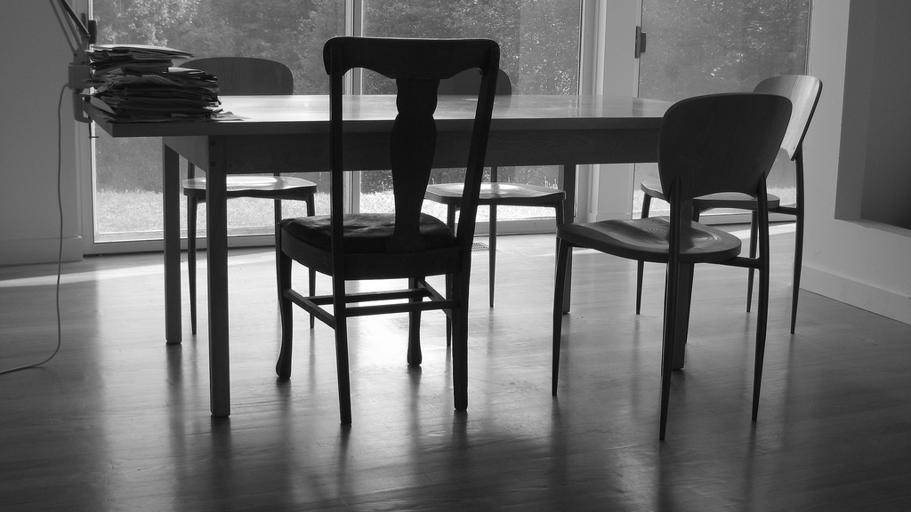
[
  {"left": 177, "top": 56, "right": 318, "bottom": 337},
  {"left": 275, "top": 36, "right": 501, "bottom": 421},
  {"left": 423, "top": 69, "right": 567, "bottom": 347},
  {"left": 551, "top": 92, "right": 793, "bottom": 442},
  {"left": 634, "top": 73, "right": 825, "bottom": 336}
]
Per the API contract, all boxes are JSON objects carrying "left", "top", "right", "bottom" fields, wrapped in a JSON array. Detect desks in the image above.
[{"left": 79, "top": 93, "right": 692, "bottom": 421}]
